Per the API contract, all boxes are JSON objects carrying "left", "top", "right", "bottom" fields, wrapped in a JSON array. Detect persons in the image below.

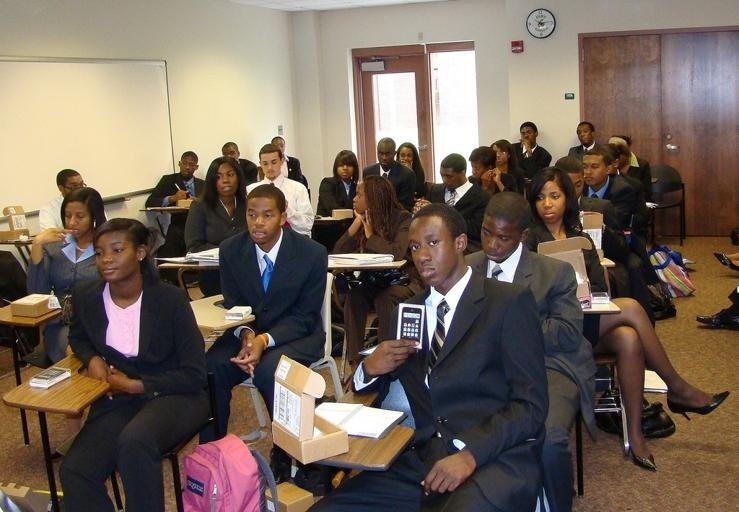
[
  {"left": 333, "top": 176, "right": 426, "bottom": 391},
  {"left": 711, "top": 250, "right": 739, "bottom": 272},
  {"left": 59, "top": 217, "right": 212, "bottom": 512},
  {"left": 554, "top": 122, "right": 655, "bottom": 326},
  {"left": 305, "top": 203, "right": 551, "bottom": 510},
  {"left": 199, "top": 185, "right": 328, "bottom": 485},
  {"left": 465, "top": 193, "right": 598, "bottom": 512},
  {"left": 413, "top": 123, "right": 552, "bottom": 242},
  {"left": 148, "top": 137, "right": 314, "bottom": 295},
  {"left": 696, "top": 284, "right": 738, "bottom": 330},
  {"left": 317, "top": 138, "right": 426, "bottom": 250},
  {"left": 26, "top": 188, "right": 107, "bottom": 365},
  {"left": 305, "top": 203, "right": 551, "bottom": 512},
  {"left": 522, "top": 167, "right": 729, "bottom": 471},
  {"left": 38, "top": 169, "right": 85, "bottom": 231}
]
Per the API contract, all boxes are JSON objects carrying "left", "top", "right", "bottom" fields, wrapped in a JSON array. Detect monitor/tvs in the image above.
[
  {"left": 28, "top": 366, "right": 71, "bottom": 390},
  {"left": 10, "top": 293, "right": 58, "bottom": 318}
]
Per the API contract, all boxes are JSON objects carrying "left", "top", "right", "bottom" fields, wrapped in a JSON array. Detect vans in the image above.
[
  {"left": 666, "top": 390, "right": 731, "bottom": 421},
  {"left": 630, "top": 446, "right": 658, "bottom": 471}
]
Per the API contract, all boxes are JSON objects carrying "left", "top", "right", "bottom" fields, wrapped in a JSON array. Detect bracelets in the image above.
[{"left": 260, "top": 334, "right": 267, "bottom": 349}]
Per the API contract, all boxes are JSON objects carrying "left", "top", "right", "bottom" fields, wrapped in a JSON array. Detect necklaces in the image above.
[{"left": 75, "top": 244, "right": 88, "bottom": 253}]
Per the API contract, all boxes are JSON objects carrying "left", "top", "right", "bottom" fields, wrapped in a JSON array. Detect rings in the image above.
[{"left": 174, "top": 184, "right": 181, "bottom": 191}]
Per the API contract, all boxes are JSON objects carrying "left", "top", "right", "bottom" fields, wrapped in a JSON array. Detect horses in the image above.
[
  {"left": 34, "top": 368, "right": 66, "bottom": 381},
  {"left": 401, "top": 308, "right": 421, "bottom": 344},
  {"left": 592, "top": 292, "right": 607, "bottom": 301}
]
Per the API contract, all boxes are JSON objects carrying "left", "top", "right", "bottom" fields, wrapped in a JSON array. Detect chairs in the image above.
[{"left": 0, "top": 161, "right": 689, "bottom": 512}]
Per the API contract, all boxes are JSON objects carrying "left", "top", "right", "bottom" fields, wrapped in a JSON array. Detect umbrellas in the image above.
[
  {"left": 270, "top": 451, "right": 290, "bottom": 483},
  {"left": 696, "top": 252, "right": 739, "bottom": 330}
]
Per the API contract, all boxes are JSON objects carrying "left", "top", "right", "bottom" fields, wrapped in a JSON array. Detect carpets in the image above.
[
  {"left": 184, "top": 182, "right": 194, "bottom": 197},
  {"left": 261, "top": 255, "right": 274, "bottom": 295},
  {"left": 426, "top": 299, "right": 450, "bottom": 387},
  {"left": 492, "top": 265, "right": 502, "bottom": 281},
  {"left": 446, "top": 191, "right": 456, "bottom": 208}
]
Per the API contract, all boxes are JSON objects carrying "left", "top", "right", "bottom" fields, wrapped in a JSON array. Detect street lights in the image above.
[
  {"left": 594, "top": 388, "right": 676, "bottom": 439},
  {"left": 295, "top": 394, "right": 337, "bottom": 496},
  {"left": 336, "top": 269, "right": 411, "bottom": 293},
  {"left": 643, "top": 244, "right": 696, "bottom": 320},
  {"left": 182, "top": 433, "right": 280, "bottom": 512}
]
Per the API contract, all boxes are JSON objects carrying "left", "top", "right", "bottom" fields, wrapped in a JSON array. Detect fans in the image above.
[{"left": 0, "top": 55, "right": 176, "bottom": 223}]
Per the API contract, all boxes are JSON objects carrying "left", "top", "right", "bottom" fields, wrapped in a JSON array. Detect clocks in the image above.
[{"left": 526, "top": 8, "right": 556, "bottom": 39}]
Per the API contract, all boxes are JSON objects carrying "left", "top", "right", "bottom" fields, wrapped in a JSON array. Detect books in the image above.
[
  {"left": 10, "top": 293, "right": 58, "bottom": 318},
  {"left": 315, "top": 402, "right": 408, "bottom": 439},
  {"left": 28, "top": 366, "right": 71, "bottom": 390}
]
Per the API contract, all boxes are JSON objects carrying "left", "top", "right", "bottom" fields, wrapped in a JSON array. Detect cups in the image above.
[{"left": 64, "top": 183, "right": 86, "bottom": 190}]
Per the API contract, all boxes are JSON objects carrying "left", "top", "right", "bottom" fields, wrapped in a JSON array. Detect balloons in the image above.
[{"left": 526, "top": 8, "right": 556, "bottom": 39}]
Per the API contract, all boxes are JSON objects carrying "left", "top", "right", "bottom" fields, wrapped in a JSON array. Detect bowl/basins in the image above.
[{"left": 75, "top": 244, "right": 88, "bottom": 253}]
